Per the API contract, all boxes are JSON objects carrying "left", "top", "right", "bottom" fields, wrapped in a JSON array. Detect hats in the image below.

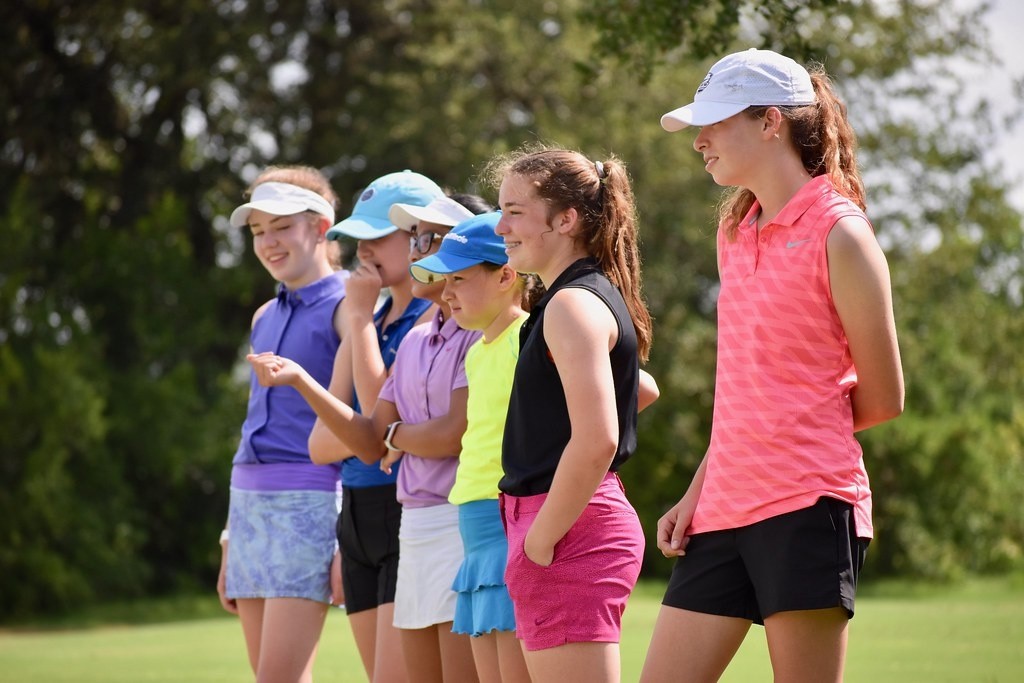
[
  {"left": 389, "top": 195, "right": 475, "bottom": 233},
  {"left": 409, "top": 210, "right": 509, "bottom": 285},
  {"left": 230, "top": 181, "right": 335, "bottom": 227},
  {"left": 661, "top": 47, "right": 817, "bottom": 132},
  {"left": 325, "top": 169, "right": 445, "bottom": 240}
]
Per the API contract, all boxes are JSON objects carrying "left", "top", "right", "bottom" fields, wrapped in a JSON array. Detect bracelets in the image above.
[{"left": 220, "top": 529, "right": 228, "bottom": 546}]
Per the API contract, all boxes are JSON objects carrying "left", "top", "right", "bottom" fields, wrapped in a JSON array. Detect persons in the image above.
[
  {"left": 216, "top": 148, "right": 660, "bottom": 683},
  {"left": 639, "top": 48, "right": 905, "bottom": 683}
]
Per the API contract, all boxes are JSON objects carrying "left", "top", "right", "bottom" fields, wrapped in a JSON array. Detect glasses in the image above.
[{"left": 409, "top": 232, "right": 443, "bottom": 253}]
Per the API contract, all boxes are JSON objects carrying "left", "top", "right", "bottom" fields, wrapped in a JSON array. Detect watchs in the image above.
[{"left": 382, "top": 421, "right": 403, "bottom": 451}]
